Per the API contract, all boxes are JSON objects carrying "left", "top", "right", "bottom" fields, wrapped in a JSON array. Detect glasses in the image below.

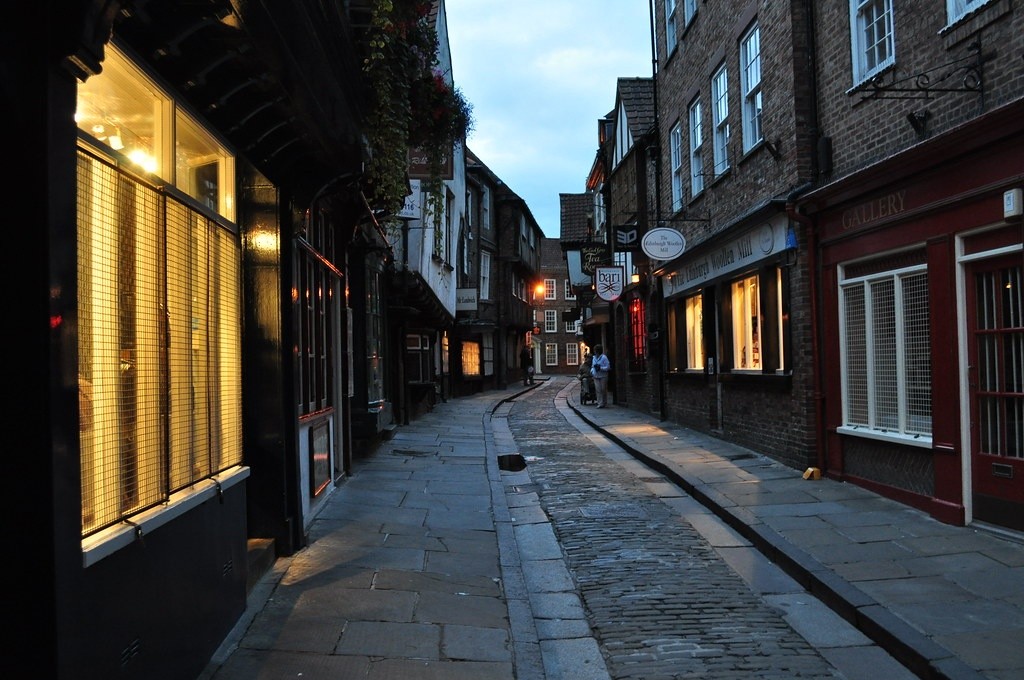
[{"left": 587, "top": 356, "right": 592, "bottom": 358}]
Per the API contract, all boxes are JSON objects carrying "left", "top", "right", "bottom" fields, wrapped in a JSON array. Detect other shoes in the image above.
[
  {"left": 525, "top": 384, "right": 530, "bottom": 386},
  {"left": 531, "top": 383, "right": 536, "bottom": 385},
  {"left": 596, "top": 403, "right": 608, "bottom": 409},
  {"left": 585, "top": 392, "right": 590, "bottom": 398}
]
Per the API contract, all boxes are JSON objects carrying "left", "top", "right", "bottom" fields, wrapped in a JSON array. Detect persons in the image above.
[
  {"left": 590, "top": 345, "right": 610, "bottom": 409},
  {"left": 577, "top": 353, "right": 597, "bottom": 401},
  {"left": 519, "top": 345, "right": 537, "bottom": 386}
]
